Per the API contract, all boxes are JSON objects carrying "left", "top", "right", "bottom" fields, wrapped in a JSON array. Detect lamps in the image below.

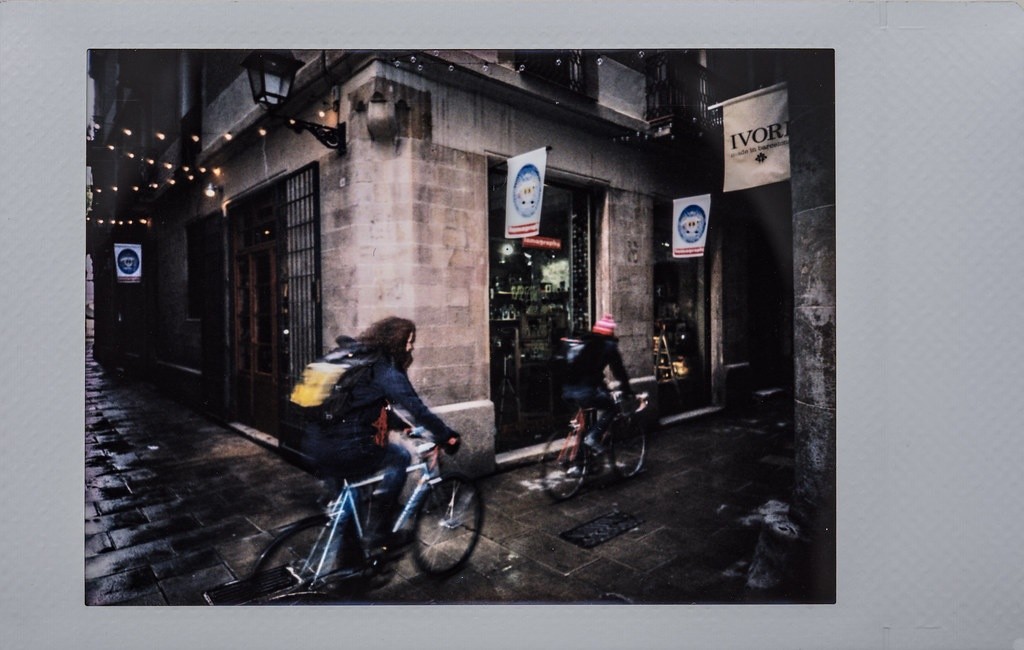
[
  {"left": 205, "top": 183, "right": 223, "bottom": 196},
  {"left": 241, "top": 49, "right": 346, "bottom": 157}
]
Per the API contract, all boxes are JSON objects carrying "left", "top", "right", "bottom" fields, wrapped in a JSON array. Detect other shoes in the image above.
[{"left": 584, "top": 434, "right": 606, "bottom": 455}]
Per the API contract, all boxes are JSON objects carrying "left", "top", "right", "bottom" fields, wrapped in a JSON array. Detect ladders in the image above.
[{"left": 652, "top": 322, "right": 681, "bottom": 397}]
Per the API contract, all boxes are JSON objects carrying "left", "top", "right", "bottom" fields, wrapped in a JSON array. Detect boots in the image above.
[{"left": 364, "top": 489, "right": 416, "bottom": 557}]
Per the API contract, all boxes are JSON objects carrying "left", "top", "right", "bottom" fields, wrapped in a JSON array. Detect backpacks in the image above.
[
  {"left": 286, "top": 357, "right": 369, "bottom": 434},
  {"left": 544, "top": 337, "right": 588, "bottom": 382}
]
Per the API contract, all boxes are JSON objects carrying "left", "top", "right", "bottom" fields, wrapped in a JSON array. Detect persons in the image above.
[
  {"left": 312, "top": 317, "right": 461, "bottom": 583},
  {"left": 558, "top": 312, "right": 636, "bottom": 474}
]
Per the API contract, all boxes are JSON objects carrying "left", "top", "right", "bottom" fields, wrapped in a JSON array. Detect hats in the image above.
[{"left": 592, "top": 313, "right": 616, "bottom": 336}]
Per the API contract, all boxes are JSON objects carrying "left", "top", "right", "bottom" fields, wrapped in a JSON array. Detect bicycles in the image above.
[
  {"left": 254, "top": 424, "right": 485, "bottom": 605},
  {"left": 540, "top": 378, "right": 648, "bottom": 501}
]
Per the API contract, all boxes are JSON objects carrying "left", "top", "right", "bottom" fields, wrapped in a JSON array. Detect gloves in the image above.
[{"left": 442, "top": 432, "right": 462, "bottom": 456}]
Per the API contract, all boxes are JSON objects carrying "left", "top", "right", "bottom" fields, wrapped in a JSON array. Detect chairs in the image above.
[{"left": 512, "top": 328, "right": 556, "bottom": 420}]
